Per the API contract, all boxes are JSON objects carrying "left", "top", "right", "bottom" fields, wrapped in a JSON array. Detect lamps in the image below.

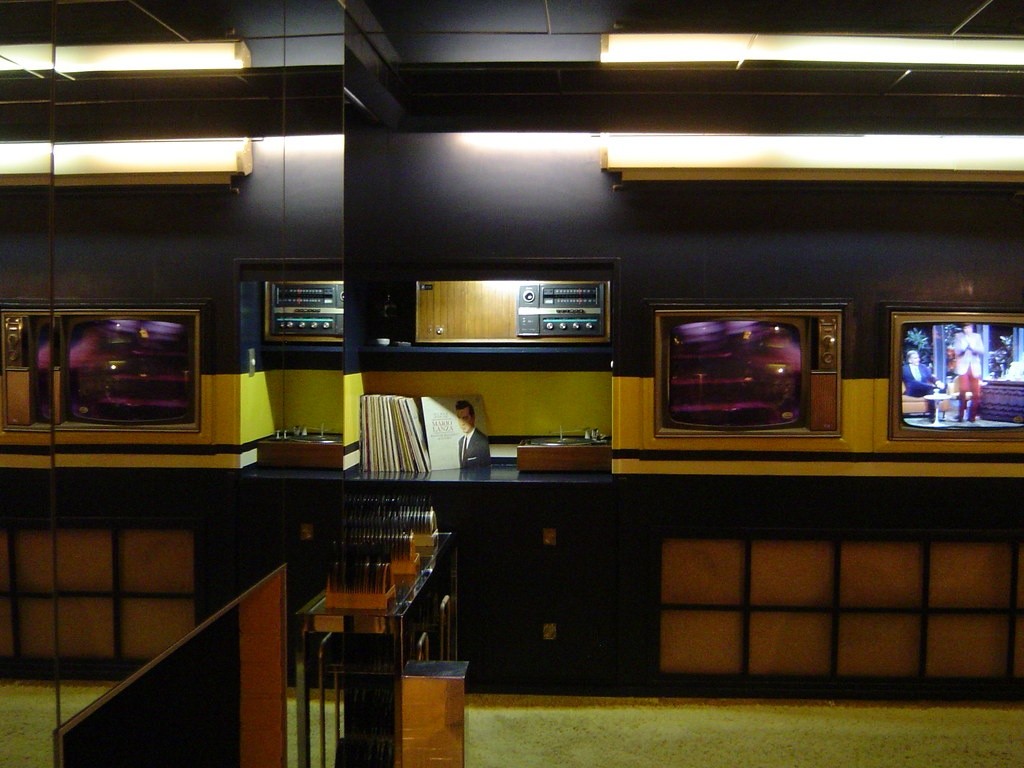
[
  {"left": 0, "top": 140, "right": 52, "bottom": 186},
  {"left": 0, "top": 41, "right": 55, "bottom": 79},
  {"left": 600, "top": 31, "right": 1024, "bottom": 73},
  {"left": 55, "top": 36, "right": 253, "bottom": 84},
  {"left": 53, "top": 136, "right": 254, "bottom": 187},
  {"left": 599, "top": 128, "right": 1024, "bottom": 182}
]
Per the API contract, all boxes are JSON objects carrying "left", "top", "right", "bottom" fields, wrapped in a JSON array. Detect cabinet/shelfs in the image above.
[{"left": 344, "top": 256, "right": 1024, "bottom": 706}]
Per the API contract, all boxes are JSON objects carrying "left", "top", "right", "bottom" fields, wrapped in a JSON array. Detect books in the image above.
[{"left": 360, "top": 393, "right": 490, "bottom": 473}]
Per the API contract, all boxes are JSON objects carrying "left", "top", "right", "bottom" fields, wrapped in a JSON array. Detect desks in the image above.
[{"left": 294, "top": 533, "right": 457, "bottom": 768}]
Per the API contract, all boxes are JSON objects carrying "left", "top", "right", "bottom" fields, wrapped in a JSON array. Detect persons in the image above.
[
  {"left": 954, "top": 324, "right": 983, "bottom": 422},
  {"left": 902, "top": 351, "right": 944, "bottom": 423},
  {"left": 454, "top": 400, "right": 489, "bottom": 468}
]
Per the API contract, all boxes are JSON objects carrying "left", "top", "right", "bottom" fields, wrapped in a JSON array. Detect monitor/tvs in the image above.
[
  {"left": 53, "top": 308, "right": 200, "bottom": 433},
  {"left": 654, "top": 309, "right": 843, "bottom": 438},
  {"left": 888, "top": 312, "right": 1024, "bottom": 442}
]
